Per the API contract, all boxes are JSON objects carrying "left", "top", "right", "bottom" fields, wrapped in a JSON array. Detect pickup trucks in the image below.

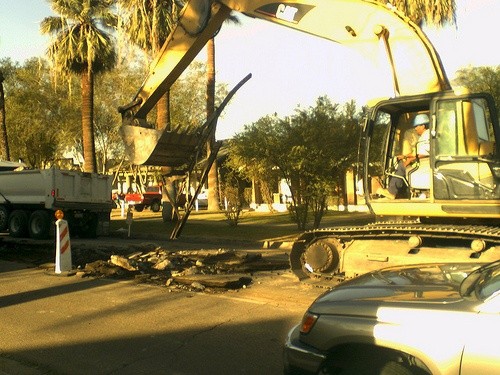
[{"left": 124, "top": 185, "right": 193, "bottom": 213}]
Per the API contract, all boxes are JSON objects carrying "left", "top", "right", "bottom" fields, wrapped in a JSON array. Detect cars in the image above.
[{"left": 282, "top": 258, "right": 500, "bottom": 375}]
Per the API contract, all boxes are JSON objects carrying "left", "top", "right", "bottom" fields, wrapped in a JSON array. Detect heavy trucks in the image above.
[{"left": 0, "top": 168, "right": 118, "bottom": 241}]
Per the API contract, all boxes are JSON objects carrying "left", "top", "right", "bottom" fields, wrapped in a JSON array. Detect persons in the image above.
[
  {"left": 376, "top": 114, "right": 430, "bottom": 200},
  {"left": 112, "top": 192, "right": 124, "bottom": 209}
]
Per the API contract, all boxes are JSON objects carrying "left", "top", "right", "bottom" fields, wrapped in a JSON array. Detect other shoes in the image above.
[{"left": 379, "top": 188, "right": 396, "bottom": 200}]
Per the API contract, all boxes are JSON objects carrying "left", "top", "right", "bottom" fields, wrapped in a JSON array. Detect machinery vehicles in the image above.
[{"left": 117, "top": 1, "right": 500, "bottom": 292}]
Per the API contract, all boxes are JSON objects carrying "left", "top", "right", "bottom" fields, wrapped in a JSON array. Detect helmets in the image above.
[{"left": 413, "top": 114, "right": 430, "bottom": 127}]
